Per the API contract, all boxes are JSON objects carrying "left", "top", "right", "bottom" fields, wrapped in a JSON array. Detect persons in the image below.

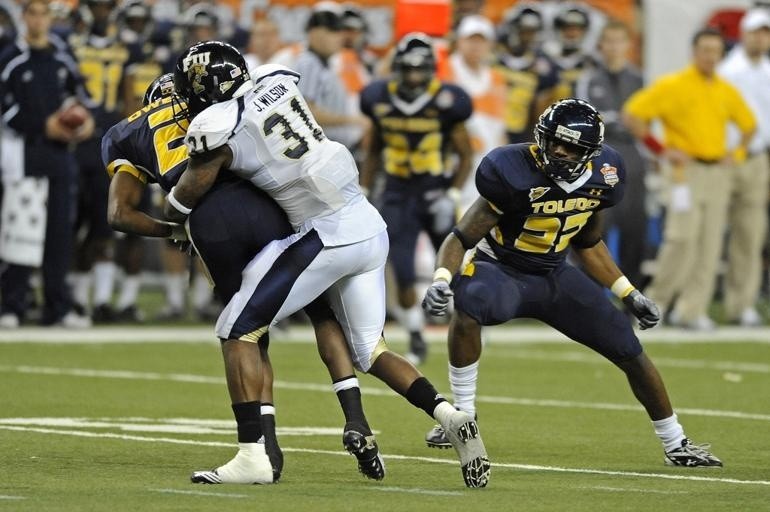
[
  {"left": 159, "top": 39, "right": 493, "bottom": 492},
  {"left": 420, "top": 97, "right": 727, "bottom": 472},
  {"left": 97, "top": 70, "right": 387, "bottom": 483}
]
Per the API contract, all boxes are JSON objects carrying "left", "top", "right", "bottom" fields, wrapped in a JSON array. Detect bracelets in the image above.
[
  {"left": 433, "top": 266, "right": 452, "bottom": 284},
  {"left": 609, "top": 276, "right": 635, "bottom": 300}
]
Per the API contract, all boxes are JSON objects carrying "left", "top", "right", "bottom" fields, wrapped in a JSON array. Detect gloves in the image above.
[
  {"left": 422, "top": 278, "right": 455, "bottom": 316},
  {"left": 624, "top": 287, "right": 661, "bottom": 331}
]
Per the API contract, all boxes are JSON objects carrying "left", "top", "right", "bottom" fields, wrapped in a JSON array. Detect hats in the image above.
[
  {"left": 303, "top": 11, "right": 350, "bottom": 33},
  {"left": 454, "top": 14, "right": 495, "bottom": 41},
  {"left": 739, "top": 7, "right": 770, "bottom": 34}
]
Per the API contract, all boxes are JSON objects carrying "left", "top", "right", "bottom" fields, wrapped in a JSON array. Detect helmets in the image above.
[
  {"left": 531, "top": 98, "right": 604, "bottom": 171},
  {"left": 551, "top": 8, "right": 589, "bottom": 41},
  {"left": 144, "top": 5, "right": 181, "bottom": 46},
  {"left": 140, "top": 70, "right": 178, "bottom": 104},
  {"left": 391, "top": 31, "right": 439, "bottom": 74},
  {"left": 173, "top": 40, "right": 252, "bottom": 102},
  {"left": 116, "top": 6, "right": 150, "bottom": 44},
  {"left": 512, "top": 9, "right": 544, "bottom": 40},
  {"left": 342, "top": 11, "right": 369, "bottom": 48},
  {"left": 183, "top": 5, "right": 219, "bottom": 39}
]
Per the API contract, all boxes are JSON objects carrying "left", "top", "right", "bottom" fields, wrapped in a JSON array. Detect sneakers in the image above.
[
  {"left": 0, "top": 292, "right": 237, "bottom": 334},
  {"left": 422, "top": 418, "right": 455, "bottom": 451},
  {"left": 260, "top": 432, "right": 285, "bottom": 480},
  {"left": 444, "top": 412, "right": 491, "bottom": 490},
  {"left": 188, "top": 442, "right": 274, "bottom": 488},
  {"left": 669, "top": 303, "right": 765, "bottom": 332},
  {"left": 662, "top": 435, "right": 722, "bottom": 469},
  {"left": 338, "top": 422, "right": 387, "bottom": 481}
]
